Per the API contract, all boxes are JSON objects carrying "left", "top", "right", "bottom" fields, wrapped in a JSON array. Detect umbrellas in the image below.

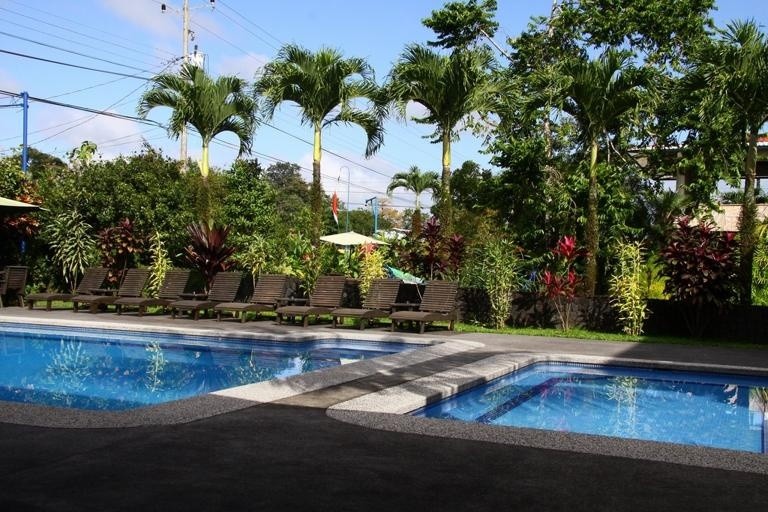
[
  {"left": 319, "top": 230, "right": 392, "bottom": 247},
  {"left": 0, "top": 196, "right": 51, "bottom": 215}
]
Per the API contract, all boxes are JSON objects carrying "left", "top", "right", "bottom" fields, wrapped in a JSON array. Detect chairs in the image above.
[
  {"left": 330, "top": 277, "right": 402, "bottom": 329},
  {"left": 274, "top": 275, "right": 348, "bottom": 328},
  {"left": 0, "top": 266, "right": 32, "bottom": 307},
  {"left": 112, "top": 269, "right": 196, "bottom": 317},
  {"left": 166, "top": 271, "right": 244, "bottom": 320},
  {"left": 22, "top": 269, "right": 109, "bottom": 310},
  {"left": 390, "top": 279, "right": 458, "bottom": 332},
  {"left": 214, "top": 274, "right": 293, "bottom": 324},
  {"left": 70, "top": 268, "right": 153, "bottom": 314}
]
